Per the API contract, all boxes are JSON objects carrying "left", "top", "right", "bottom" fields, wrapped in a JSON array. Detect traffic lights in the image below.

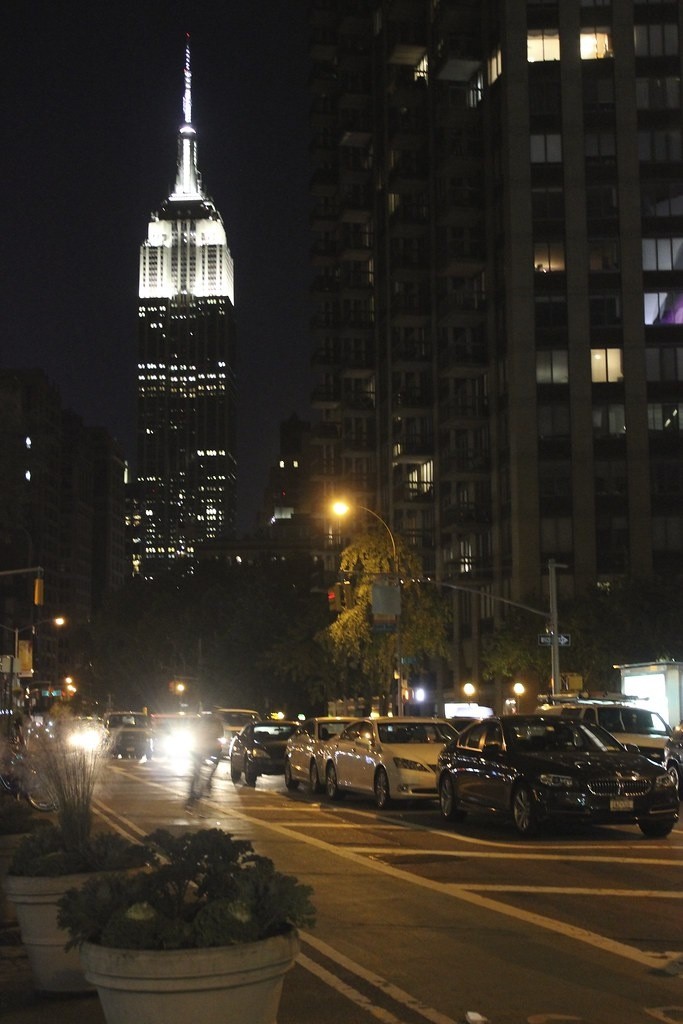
[{"left": 328, "top": 585, "right": 342, "bottom": 612}]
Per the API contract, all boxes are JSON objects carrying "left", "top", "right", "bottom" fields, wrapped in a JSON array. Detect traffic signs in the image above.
[{"left": 537, "top": 634, "right": 572, "bottom": 647}]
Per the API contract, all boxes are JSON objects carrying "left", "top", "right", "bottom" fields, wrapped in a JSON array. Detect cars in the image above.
[
  {"left": 436, "top": 713, "right": 681, "bottom": 841},
  {"left": 230, "top": 719, "right": 302, "bottom": 788},
  {"left": 284, "top": 716, "right": 370, "bottom": 794},
  {"left": 321, "top": 715, "right": 463, "bottom": 812},
  {"left": 23, "top": 702, "right": 265, "bottom": 759},
  {"left": 663, "top": 720, "right": 683, "bottom": 802}
]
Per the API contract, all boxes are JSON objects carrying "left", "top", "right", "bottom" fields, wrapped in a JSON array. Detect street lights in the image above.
[
  {"left": 330, "top": 494, "right": 404, "bottom": 719},
  {"left": 1, "top": 615, "right": 67, "bottom": 737},
  {"left": 171, "top": 673, "right": 204, "bottom": 714}
]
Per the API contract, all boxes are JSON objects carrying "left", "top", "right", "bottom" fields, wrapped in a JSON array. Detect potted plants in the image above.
[
  {"left": 0, "top": 803, "right": 63, "bottom": 921},
  {"left": 2, "top": 834, "right": 160, "bottom": 992},
  {"left": 56, "top": 827, "right": 317, "bottom": 1024}
]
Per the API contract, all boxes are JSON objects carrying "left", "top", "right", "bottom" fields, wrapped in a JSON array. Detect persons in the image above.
[
  {"left": 365, "top": 700, "right": 395, "bottom": 718},
  {"left": 563, "top": 728, "right": 585, "bottom": 748},
  {"left": 408, "top": 730, "right": 435, "bottom": 744},
  {"left": 503, "top": 698, "right": 518, "bottom": 716},
  {"left": 192, "top": 704, "right": 224, "bottom": 767},
  {"left": 629, "top": 713, "right": 639, "bottom": 725}
]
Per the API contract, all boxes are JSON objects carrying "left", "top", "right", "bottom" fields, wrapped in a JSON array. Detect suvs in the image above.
[{"left": 533, "top": 689, "right": 672, "bottom": 764}]
